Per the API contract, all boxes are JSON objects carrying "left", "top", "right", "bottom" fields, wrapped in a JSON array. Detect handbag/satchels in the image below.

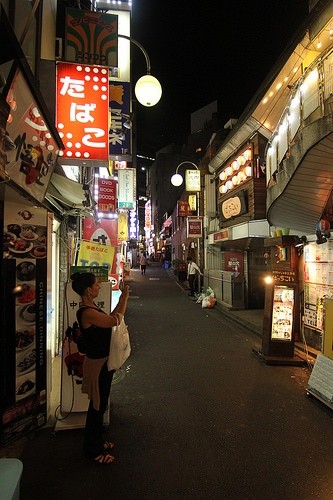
[{"left": 107, "top": 312, "right": 131, "bottom": 372}]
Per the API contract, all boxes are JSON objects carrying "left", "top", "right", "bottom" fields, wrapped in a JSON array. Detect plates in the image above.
[
  {"left": 3, "top": 232, "right": 17, "bottom": 245},
  {"left": 16, "top": 262, "right": 37, "bottom": 281},
  {"left": 15, "top": 380, "right": 36, "bottom": 401},
  {"left": 8, "top": 237, "right": 33, "bottom": 253},
  {"left": 18, "top": 306, "right": 37, "bottom": 325},
  {"left": 16, "top": 354, "right": 36, "bottom": 375},
  {"left": 20, "top": 231, "right": 39, "bottom": 241},
  {"left": 30, "top": 245, "right": 46, "bottom": 259},
  {"left": 16, "top": 328, "right": 35, "bottom": 351},
  {"left": 15, "top": 289, "right": 36, "bottom": 305}
]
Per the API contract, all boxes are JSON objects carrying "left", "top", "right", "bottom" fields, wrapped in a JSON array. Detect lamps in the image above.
[
  {"left": 118, "top": 34, "right": 162, "bottom": 107},
  {"left": 109, "top": 126, "right": 127, "bottom": 140},
  {"left": 294, "top": 234, "right": 306, "bottom": 248},
  {"left": 315, "top": 230, "right": 330, "bottom": 244},
  {"left": 171, "top": 161, "right": 198, "bottom": 187},
  {"left": 109, "top": 110, "right": 134, "bottom": 128}
]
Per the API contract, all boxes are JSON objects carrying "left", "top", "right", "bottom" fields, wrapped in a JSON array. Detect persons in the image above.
[
  {"left": 186, "top": 256, "right": 203, "bottom": 296},
  {"left": 143, "top": 248, "right": 146, "bottom": 258},
  {"left": 150, "top": 252, "right": 155, "bottom": 261},
  {"left": 69, "top": 269, "right": 130, "bottom": 464},
  {"left": 139, "top": 255, "right": 147, "bottom": 275}
]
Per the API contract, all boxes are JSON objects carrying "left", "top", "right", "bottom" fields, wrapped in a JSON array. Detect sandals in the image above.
[{"left": 87, "top": 439, "right": 119, "bottom": 467}]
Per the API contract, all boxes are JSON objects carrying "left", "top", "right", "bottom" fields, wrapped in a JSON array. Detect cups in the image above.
[
  {"left": 271, "top": 231, "right": 276, "bottom": 237},
  {"left": 276, "top": 229, "right": 282, "bottom": 236},
  {"left": 282, "top": 228, "right": 290, "bottom": 235}
]
